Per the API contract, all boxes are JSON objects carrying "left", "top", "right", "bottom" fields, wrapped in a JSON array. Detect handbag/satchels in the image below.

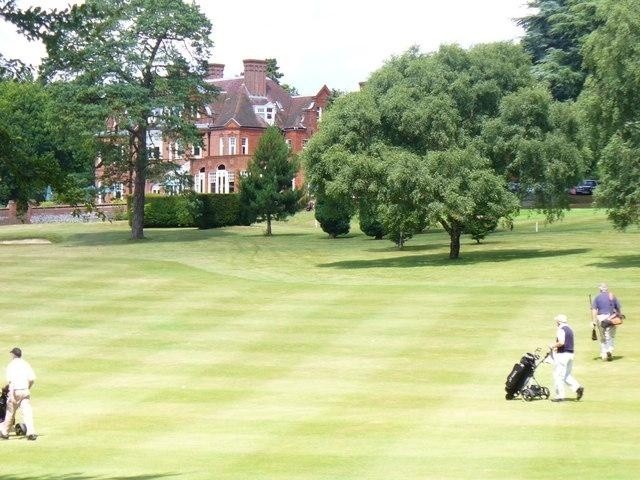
[{"left": 610, "top": 315, "right": 623, "bottom": 325}]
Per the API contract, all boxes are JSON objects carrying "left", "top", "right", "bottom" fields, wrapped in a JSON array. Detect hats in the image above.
[
  {"left": 600, "top": 283, "right": 608, "bottom": 291},
  {"left": 555, "top": 315, "right": 567, "bottom": 322},
  {"left": 10, "top": 347, "right": 21, "bottom": 356}
]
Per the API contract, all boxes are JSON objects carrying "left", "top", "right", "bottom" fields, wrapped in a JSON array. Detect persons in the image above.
[
  {"left": 591, "top": 282, "right": 621, "bottom": 362},
  {"left": 0, "top": 347, "right": 40, "bottom": 441},
  {"left": 544, "top": 313, "right": 584, "bottom": 402}
]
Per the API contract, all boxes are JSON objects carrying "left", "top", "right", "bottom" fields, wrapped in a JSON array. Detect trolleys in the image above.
[
  {"left": 504, "top": 344, "right": 554, "bottom": 401},
  {"left": 0, "top": 384, "right": 27, "bottom": 436}
]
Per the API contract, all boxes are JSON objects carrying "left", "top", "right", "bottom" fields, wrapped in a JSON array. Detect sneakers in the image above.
[
  {"left": 577, "top": 387, "right": 583, "bottom": 400},
  {"left": 29, "top": 435, "right": 36, "bottom": 440},
  {"left": 0, "top": 432, "right": 8, "bottom": 439},
  {"left": 551, "top": 398, "right": 562, "bottom": 402},
  {"left": 607, "top": 351, "right": 612, "bottom": 361}
]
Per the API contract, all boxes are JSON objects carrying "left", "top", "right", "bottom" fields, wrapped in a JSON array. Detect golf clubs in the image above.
[
  {"left": 589, "top": 294, "right": 597, "bottom": 340},
  {"left": 533, "top": 348, "right": 541, "bottom": 360}
]
